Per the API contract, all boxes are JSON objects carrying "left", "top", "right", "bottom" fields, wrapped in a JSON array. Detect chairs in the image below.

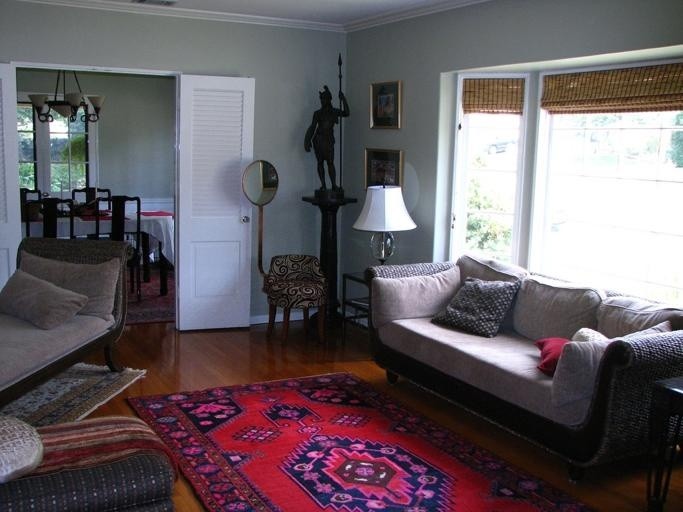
[
  {"left": 20, "top": 184, "right": 171, "bottom": 303},
  {"left": 260, "top": 253, "right": 328, "bottom": 344}
]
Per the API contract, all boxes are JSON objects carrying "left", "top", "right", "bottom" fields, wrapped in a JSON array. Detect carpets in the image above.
[
  {"left": 1, "top": 361, "right": 149, "bottom": 425},
  {"left": 125, "top": 369, "right": 608, "bottom": 510}
]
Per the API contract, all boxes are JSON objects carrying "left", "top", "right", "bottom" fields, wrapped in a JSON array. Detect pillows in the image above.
[
  {"left": 369, "top": 265, "right": 461, "bottom": 325},
  {"left": 0, "top": 417, "right": 40, "bottom": 477},
  {"left": 429, "top": 270, "right": 518, "bottom": 337},
  {"left": 534, "top": 333, "right": 576, "bottom": 376},
  {"left": 591, "top": 293, "right": 683, "bottom": 334},
  {"left": 549, "top": 318, "right": 673, "bottom": 408},
  {"left": 512, "top": 277, "right": 604, "bottom": 347},
  {"left": 453, "top": 252, "right": 522, "bottom": 291},
  {"left": 14, "top": 248, "right": 122, "bottom": 317},
  {"left": 0, "top": 269, "right": 88, "bottom": 328}
]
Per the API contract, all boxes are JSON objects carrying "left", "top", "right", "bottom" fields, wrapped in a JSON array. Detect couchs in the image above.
[
  {"left": 366, "top": 255, "right": 683, "bottom": 485},
  {"left": 0, "top": 235, "right": 127, "bottom": 405},
  {"left": 0, "top": 410, "right": 177, "bottom": 509}
]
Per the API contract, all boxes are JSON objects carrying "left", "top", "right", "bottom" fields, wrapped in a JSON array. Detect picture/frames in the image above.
[
  {"left": 368, "top": 79, "right": 401, "bottom": 129},
  {"left": 363, "top": 145, "right": 402, "bottom": 195}
]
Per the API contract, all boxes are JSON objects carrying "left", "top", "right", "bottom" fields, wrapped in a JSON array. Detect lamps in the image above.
[
  {"left": 26, "top": 75, "right": 106, "bottom": 123},
  {"left": 352, "top": 178, "right": 419, "bottom": 265}
]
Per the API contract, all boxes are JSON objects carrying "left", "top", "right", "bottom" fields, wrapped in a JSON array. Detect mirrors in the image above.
[{"left": 240, "top": 159, "right": 281, "bottom": 277}]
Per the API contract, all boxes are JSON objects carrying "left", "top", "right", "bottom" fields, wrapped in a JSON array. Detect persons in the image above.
[{"left": 304, "top": 91, "right": 351, "bottom": 191}]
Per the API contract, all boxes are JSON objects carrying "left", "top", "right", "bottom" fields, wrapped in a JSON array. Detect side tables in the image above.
[
  {"left": 645, "top": 373, "right": 682, "bottom": 512},
  {"left": 341, "top": 268, "right": 374, "bottom": 331}
]
